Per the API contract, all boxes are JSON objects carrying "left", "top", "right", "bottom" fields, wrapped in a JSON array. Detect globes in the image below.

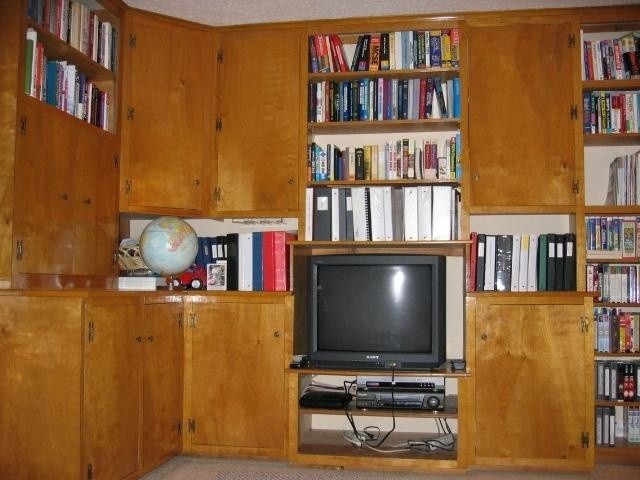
[{"left": 139, "top": 214, "right": 198, "bottom": 291}]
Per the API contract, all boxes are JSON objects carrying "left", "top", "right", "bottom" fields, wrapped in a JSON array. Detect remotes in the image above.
[{"left": 451, "top": 359, "right": 465, "bottom": 369}]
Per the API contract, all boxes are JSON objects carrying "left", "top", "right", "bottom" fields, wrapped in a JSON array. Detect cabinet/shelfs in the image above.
[
  {"left": 9, "top": 0, "right": 120, "bottom": 283},
  {"left": 123, "top": 5, "right": 304, "bottom": 217},
  {"left": 464, "top": 15, "right": 640, "bottom": 469},
  {"left": 300, "top": 368, "right": 459, "bottom": 460},
  {"left": 308, "top": 19, "right": 463, "bottom": 237},
  {"left": 5, "top": 286, "right": 293, "bottom": 480}
]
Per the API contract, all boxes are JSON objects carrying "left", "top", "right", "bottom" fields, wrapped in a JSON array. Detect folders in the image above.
[
  {"left": 195, "top": 236, "right": 227, "bottom": 272},
  {"left": 469, "top": 232, "right": 576, "bottom": 292}
]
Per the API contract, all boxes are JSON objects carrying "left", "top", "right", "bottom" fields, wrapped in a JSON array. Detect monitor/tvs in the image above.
[{"left": 309, "top": 255, "right": 446, "bottom": 370}]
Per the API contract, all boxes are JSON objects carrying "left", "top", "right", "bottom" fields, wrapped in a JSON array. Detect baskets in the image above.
[{"left": 117, "top": 244, "right": 149, "bottom": 271}]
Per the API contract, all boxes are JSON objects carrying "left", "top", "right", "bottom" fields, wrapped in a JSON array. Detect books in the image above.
[
  {"left": 576, "top": 28, "right": 640, "bottom": 447},
  {"left": 305, "top": 27, "right": 461, "bottom": 242},
  {"left": 24, "top": 0, "right": 116, "bottom": 130},
  {"left": 471, "top": 232, "right": 581, "bottom": 294},
  {"left": 192, "top": 232, "right": 298, "bottom": 293}
]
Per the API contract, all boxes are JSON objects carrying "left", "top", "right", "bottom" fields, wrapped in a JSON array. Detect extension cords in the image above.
[{"left": 427, "top": 435, "right": 454, "bottom": 451}]
